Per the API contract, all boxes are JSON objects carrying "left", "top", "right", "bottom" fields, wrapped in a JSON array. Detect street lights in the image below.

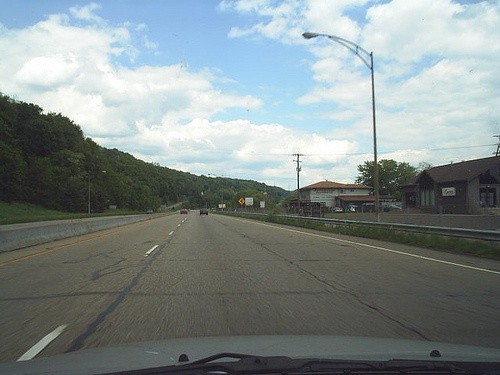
[
  {"left": 302, "top": 31, "right": 378, "bottom": 223},
  {"left": 208, "top": 173, "right": 223, "bottom": 212},
  {"left": 88, "top": 170, "right": 106, "bottom": 214}
]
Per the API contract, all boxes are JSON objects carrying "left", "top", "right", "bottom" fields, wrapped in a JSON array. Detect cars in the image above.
[
  {"left": 146, "top": 208, "right": 153, "bottom": 214},
  {"left": 331, "top": 207, "right": 344, "bottom": 213},
  {"left": 180, "top": 208, "right": 188, "bottom": 214},
  {"left": 200, "top": 208, "right": 208, "bottom": 215}
]
